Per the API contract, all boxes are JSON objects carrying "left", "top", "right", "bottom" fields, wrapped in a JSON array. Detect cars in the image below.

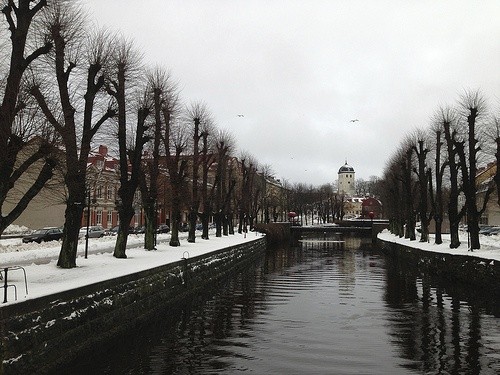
[
  {"left": 79, "top": 226, "right": 106, "bottom": 238},
  {"left": 181, "top": 223, "right": 191, "bottom": 231},
  {"left": 155, "top": 225, "right": 171, "bottom": 234},
  {"left": 22, "top": 227, "right": 64, "bottom": 244},
  {"left": 133, "top": 225, "right": 148, "bottom": 233},
  {"left": 481, "top": 225, "right": 500, "bottom": 235},
  {"left": 196, "top": 224, "right": 204, "bottom": 231},
  {"left": 208, "top": 222, "right": 217, "bottom": 229},
  {"left": 107, "top": 225, "right": 135, "bottom": 236}
]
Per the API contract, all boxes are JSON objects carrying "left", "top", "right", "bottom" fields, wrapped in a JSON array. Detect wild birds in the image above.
[
  {"left": 238, "top": 114, "right": 244, "bottom": 118},
  {"left": 350, "top": 119, "right": 358, "bottom": 122}
]
